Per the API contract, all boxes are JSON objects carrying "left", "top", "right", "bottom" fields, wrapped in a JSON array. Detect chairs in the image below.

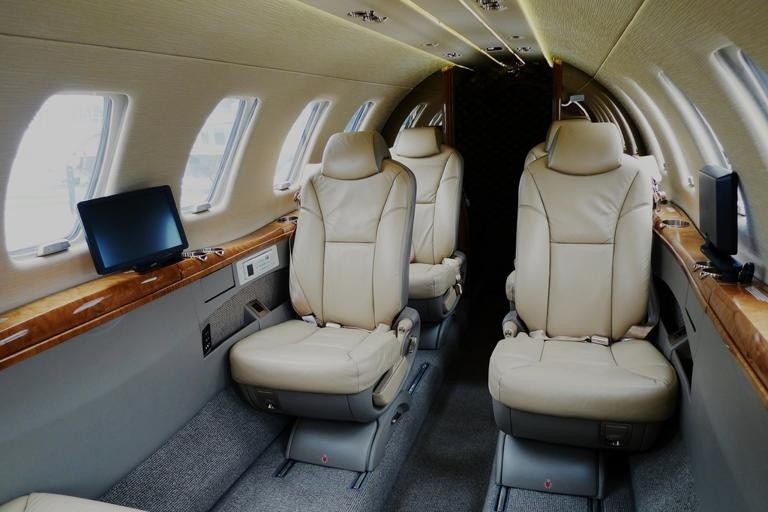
[
  {"left": 226, "top": 126, "right": 422, "bottom": 425},
  {"left": 389, "top": 123, "right": 472, "bottom": 326},
  {"left": 486, "top": 116, "right": 677, "bottom": 455}
]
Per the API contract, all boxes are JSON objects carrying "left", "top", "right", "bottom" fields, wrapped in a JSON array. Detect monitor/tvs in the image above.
[{"left": 76, "top": 185, "right": 188, "bottom": 274}]
